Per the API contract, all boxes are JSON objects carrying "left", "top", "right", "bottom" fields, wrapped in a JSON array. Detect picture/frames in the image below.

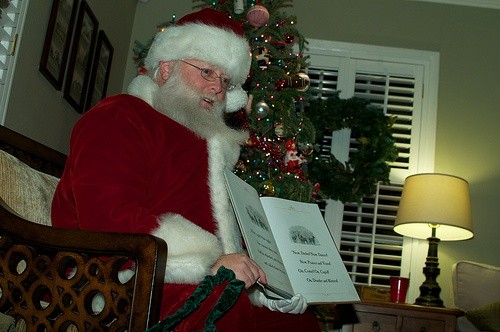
[{"left": 40, "top": 0, "right": 114, "bottom": 115}]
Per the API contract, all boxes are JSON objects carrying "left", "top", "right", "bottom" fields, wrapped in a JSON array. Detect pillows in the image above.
[{"left": 466, "top": 299, "right": 500, "bottom": 332}]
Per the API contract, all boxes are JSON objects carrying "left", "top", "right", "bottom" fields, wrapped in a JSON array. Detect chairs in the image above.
[
  {"left": 0, "top": 124, "right": 167, "bottom": 332},
  {"left": 453, "top": 260, "right": 500, "bottom": 332}
]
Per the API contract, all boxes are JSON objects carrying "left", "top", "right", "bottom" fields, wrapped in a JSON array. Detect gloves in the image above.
[{"left": 258, "top": 292, "right": 307, "bottom": 313}]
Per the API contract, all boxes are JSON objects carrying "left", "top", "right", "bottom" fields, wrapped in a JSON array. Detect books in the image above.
[{"left": 223, "top": 167, "right": 361, "bottom": 304}]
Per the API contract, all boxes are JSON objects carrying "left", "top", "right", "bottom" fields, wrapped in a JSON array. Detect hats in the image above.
[{"left": 143, "top": 7, "right": 251, "bottom": 112}]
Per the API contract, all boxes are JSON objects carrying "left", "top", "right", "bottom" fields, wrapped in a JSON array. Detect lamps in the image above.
[{"left": 393, "top": 173, "right": 475, "bottom": 308}]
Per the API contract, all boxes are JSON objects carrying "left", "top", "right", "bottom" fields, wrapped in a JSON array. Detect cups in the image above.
[{"left": 389, "top": 276, "right": 410, "bottom": 304}]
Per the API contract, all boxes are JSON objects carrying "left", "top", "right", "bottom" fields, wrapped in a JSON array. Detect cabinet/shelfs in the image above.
[{"left": 341, "top": 302, "right": 466, "bottom": 332}]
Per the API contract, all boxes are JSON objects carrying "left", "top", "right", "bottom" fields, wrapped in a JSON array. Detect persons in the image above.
[{"left": 50, "top": 9, "right": 320, "bottom": 332}]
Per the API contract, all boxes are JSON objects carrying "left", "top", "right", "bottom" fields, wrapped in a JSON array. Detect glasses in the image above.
[{"left": 179, "top": 59, "right": 235, "bottom": 90}]
